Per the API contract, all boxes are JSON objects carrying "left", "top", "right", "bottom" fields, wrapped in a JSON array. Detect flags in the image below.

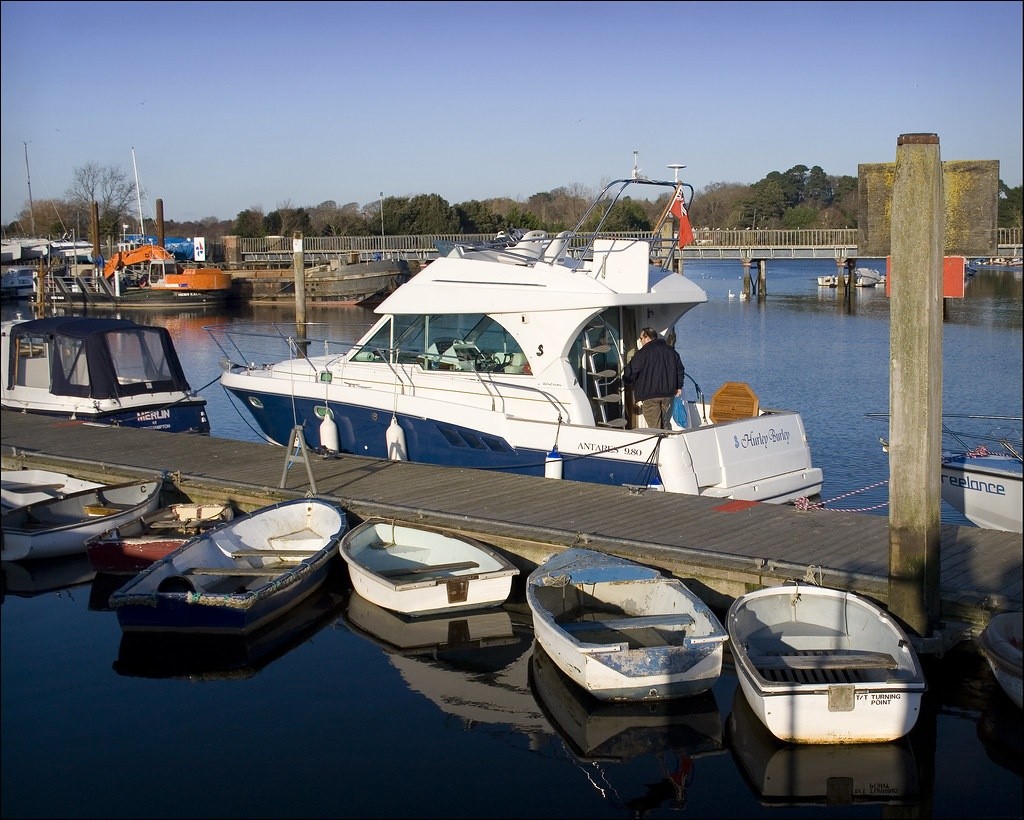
[{"left": 669, "top": 187, "right": 695, "bottom": 250}]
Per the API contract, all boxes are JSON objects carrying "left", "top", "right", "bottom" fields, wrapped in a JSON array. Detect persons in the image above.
[{"left": 623, "top": 328, "right": 685, "bottom": 430}]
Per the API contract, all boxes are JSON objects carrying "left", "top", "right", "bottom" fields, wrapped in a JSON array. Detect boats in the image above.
[
  {"left": 337, "top": 516, "right": 521, "bottom": 617},
  {"left": 202, "top": 144, "right": 826, "bottom": 505},
  {"left": 526, "top": 635, "right": 731, "bottom": 820},
  {"left": 722, "top": 684, "right": 917, "bottom": 820},
  {"left": 0, "top": 225, "right": 215, "bottom": 436},
  {"left": 0, "top": 147, "right": 235, "bottom": 311},
  {"left": 338, "top": 579, "right": 522, "bottom": 658},
  {"left": 524, "top": 546, "right": 731, "bottom": 706},
  {"left": 964, "top": 257, "right": 1023, "bottom": 276},
  {"left": 0, "top": 553, "right": 101, "bottom": 607},
  {"left": 0, "top": 470, "right": 110, "bottom": 510},
  {"left": 82, "top": 502, "right": 235, "bottom": 576},
  {"left": 430, "top": 225, "right": 533, "bottom": 258},
  {"left": 220, "top": 191, "right": 413, "bottom": 306},
  {"left": 106, "top": 496, "right": 352, "bottom": 635},
  {"left": 817, "top": 267, "right": 888, "bottom": 289},
  {"left": 862, "top": 411, "right": 1024, "bottom": 534},
  {"left": 0, "top": 472, "right": 165, "bottom": 563},
  {"left": 724, "top": 580, "right": 928, "bottom": 746},
  {"left": 978, "top": 611, "right": 1024, "bottom": 714},
  {"left": 110, "top": 561, "right": 352, "bottom": 683}
]
